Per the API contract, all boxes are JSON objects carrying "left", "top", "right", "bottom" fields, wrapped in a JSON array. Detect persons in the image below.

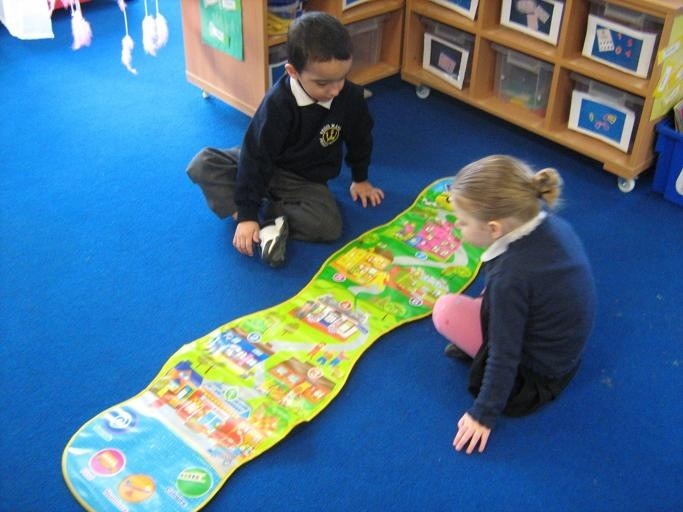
[
  {"left": 185, "top": 12, "right": 384, "bottom": 268},
  {"left": 430, "top": 154, "right": 596, "bottom": 457}
]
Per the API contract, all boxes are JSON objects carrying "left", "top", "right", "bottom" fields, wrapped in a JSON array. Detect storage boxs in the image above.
[
  {"left": 568, "top": 72, "right": 645, "bottom": 152},
  {"left": 267, "top": 42, "right": 290, "bottom": 91},
  {"left": 338, "top": 13, "right": 392, "bottom": 72},
  {"left": 420, "top": 20, "right": 475, "bottom": 81},
  {"left": 652, "top": 117, "right": 683, "bottom": 208},
  {"left": 490, "top": 42, "right": 553, "bottom": 118},
  {"left": 585, "top": 1, "right": 664, "bottom": 78}
]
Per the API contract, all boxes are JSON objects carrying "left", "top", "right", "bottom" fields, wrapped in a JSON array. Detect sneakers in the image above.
[
  {"left": 444, "top": 343, "right": 475, "bottom": 364},
  {"left": 256, "top": 216, "right": 290, "bottom": 266}
]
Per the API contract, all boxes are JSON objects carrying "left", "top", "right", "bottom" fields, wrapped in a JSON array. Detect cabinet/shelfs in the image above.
[
  {"left": 182, "top": 0, "right": 404, "bottom": 119},
  {"left": 400, "top": 0, "right": 683, "bottom": 192}
]
